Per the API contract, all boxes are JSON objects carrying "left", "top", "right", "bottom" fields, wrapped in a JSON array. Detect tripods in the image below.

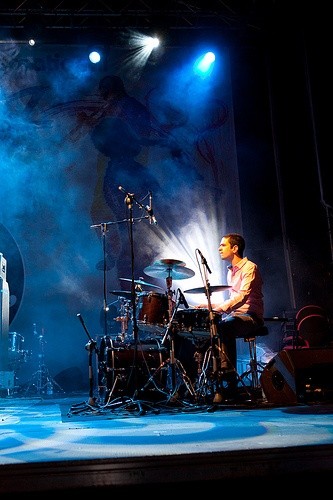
[
  {"left": 25, "top": 324, "right": 66, "bottom": 395},
  {"left": 71, "top": 186, "right": 251, "bottom": 415}
]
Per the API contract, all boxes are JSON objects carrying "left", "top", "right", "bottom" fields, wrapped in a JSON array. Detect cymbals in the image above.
[
  {"left": 143, "top": 259, "right": 195, "bottom": 280},
  {"left": 109, "top": 290, "right": 137, "bottom": 299},
  {"left": 183, "top": 285, "right": 232, "bottom": 293},
  {"left": 119, "top": 277, "right": 161, "bottom": 289}
]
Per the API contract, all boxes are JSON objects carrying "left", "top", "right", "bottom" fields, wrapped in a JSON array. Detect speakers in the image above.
[{"left": 259, "top": 346, "right": 333, "bottom": 405}]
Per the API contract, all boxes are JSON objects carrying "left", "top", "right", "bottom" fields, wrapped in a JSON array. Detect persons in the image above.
[{"left": 177, "top": 232, "right": 264, "bottom": 389}]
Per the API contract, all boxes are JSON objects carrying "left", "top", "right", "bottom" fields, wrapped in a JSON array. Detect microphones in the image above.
[
  {"left": 149, "top": 194, "right": 152, "bottom": 224},
  {"left": 178, "top": 288, "right": 189, "bottom": 309},
  {"left": 198, "top": 250, "right": 212, "bottom": 274}
]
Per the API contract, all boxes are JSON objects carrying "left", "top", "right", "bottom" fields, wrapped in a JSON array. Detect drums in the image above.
[
  {"left": 97, "top": 333, "right": 170, "bottom": 401},
  {"left": 174, "top": 308, "right": 222, "bottom": 332},
  {"left": 8, "top": 332, "right": 24, "bottom": 361},
  {"left": 133, "top": 290, "right": 169, "bottom": 326}
]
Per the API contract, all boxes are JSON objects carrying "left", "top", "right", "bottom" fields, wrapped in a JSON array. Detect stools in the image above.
[{"left": 237, "top": 326, "right": 269, "bottom": 405}]
